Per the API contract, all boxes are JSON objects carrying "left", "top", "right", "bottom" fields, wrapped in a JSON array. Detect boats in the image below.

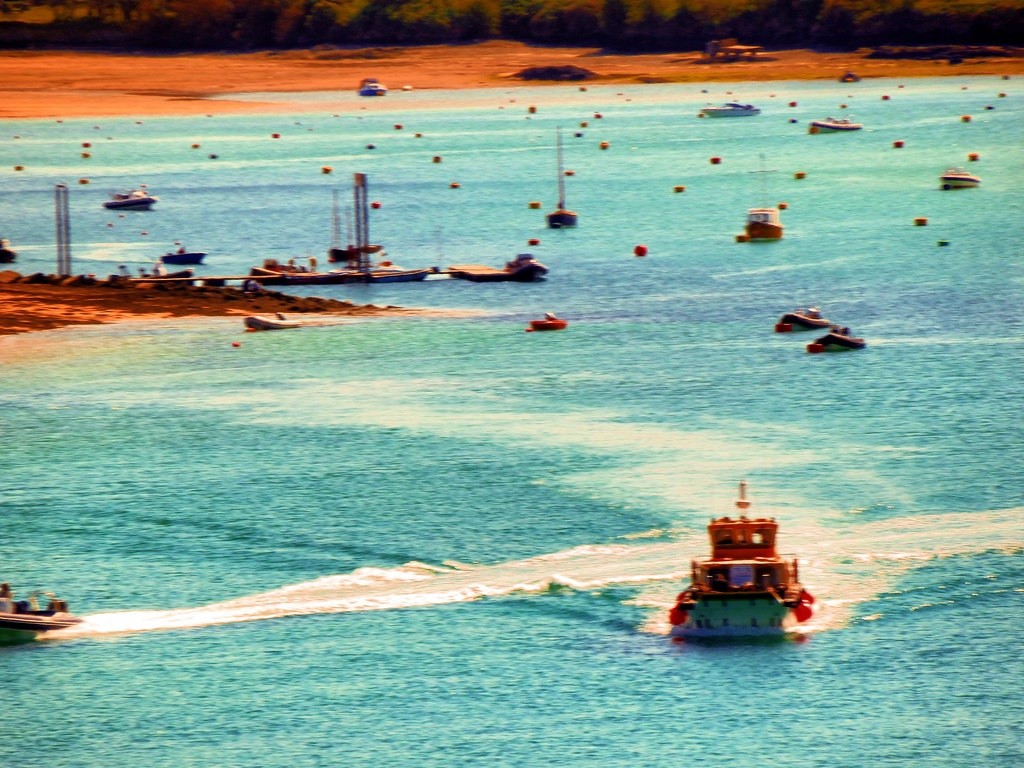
[
  {"left": 448, "top": 258, "right": 547, "bottom": 281},
  {"left": 531, "top": 319, "right": 567, "bottom": 330},
  {"left": 358, "top": 84, "right": 386, "bottom": 95},
  {"left": 701, "top": 105, "right": 759, "bottom": 117},
  {"left": 745, "top": 208, "right": 785, "bottom": 242},
  {"left": 103, "top": 196, "right": 158, "bottom": 210},
  {"left": 779, "top": 313, "right": 829, "bottom": 330},
  {"left": 839, "top": 73, "right": 860, "bottom": 81},
  {"left": 249, "top": 265, "right": 428, "bottom": 284},
  {"left": 238, "top": 316, "right": 302, "bottom": 330},
  {"left": 811, "top": 119, "right": 864, "bottom": 132},
  {"left": 939, "top": 172, "right": 981, "bottom": 187},
  {"left": 161, "top": 253, "right": 207, "bottom": 265},
  {"left": 814, "top": 333, "right": 866, "bottom": 350},
  {"left": 328, "top": 247, "right": 351, "bottom": 259},
  {"left": 670, "top": 518, "right": 808, "bottom": 635},
  {"left": 0, "top": 608, "right": 86, "bottom": 643}
]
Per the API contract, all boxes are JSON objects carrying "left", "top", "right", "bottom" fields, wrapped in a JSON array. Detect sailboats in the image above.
[{"left": 545, "top": 131, "right": 580, "bottom": 228}]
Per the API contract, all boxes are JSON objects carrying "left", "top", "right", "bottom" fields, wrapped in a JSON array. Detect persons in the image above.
[
  {"left": 0, "top": 583, "right": 12, "bottom": 598},
  {"left": 710, "top": 573, "right": 729, "bottom": 592}
]
